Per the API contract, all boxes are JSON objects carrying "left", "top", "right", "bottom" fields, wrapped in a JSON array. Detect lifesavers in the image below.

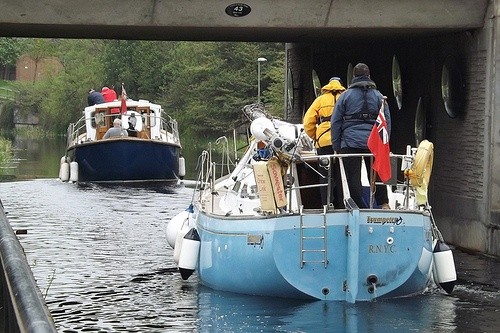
[
  {"left": 122, "top": 111, "right": 143, "bottom": 132},
  {"left": 412, "top": 140, "right": 434, "bottom": 204}
]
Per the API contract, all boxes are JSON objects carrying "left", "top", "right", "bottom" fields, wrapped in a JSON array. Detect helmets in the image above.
[{"left": 329, "top": 77, "right": 343, "bottom": 85}]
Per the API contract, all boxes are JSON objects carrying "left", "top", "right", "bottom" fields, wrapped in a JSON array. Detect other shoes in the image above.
[{"left": 382, "top": 203, "right": 390, "bottom": 209}]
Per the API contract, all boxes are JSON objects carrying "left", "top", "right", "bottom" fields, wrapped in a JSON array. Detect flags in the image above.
[
  {"left": 367, "top": 104, "right": 393, "bottom": 183},
  {"left": 120, "top": 86, "right": 128, "bottom": 114}
]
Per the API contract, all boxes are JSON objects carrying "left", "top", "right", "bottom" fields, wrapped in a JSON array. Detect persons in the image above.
[
  {"left": 303, "top": 77, "right": 352, "bottom": 211},
  {"left": 87, "top": 89, "right": 107, "bottom": 127},
  {"left": 331, "top": 62, "right": 391, "bottom": 210},
  {"left": 99, "top": 86, "right": 118, "bottom": 126},
  {"left": 102, "top": 120, "right": 128, "bottom": 140}
]
